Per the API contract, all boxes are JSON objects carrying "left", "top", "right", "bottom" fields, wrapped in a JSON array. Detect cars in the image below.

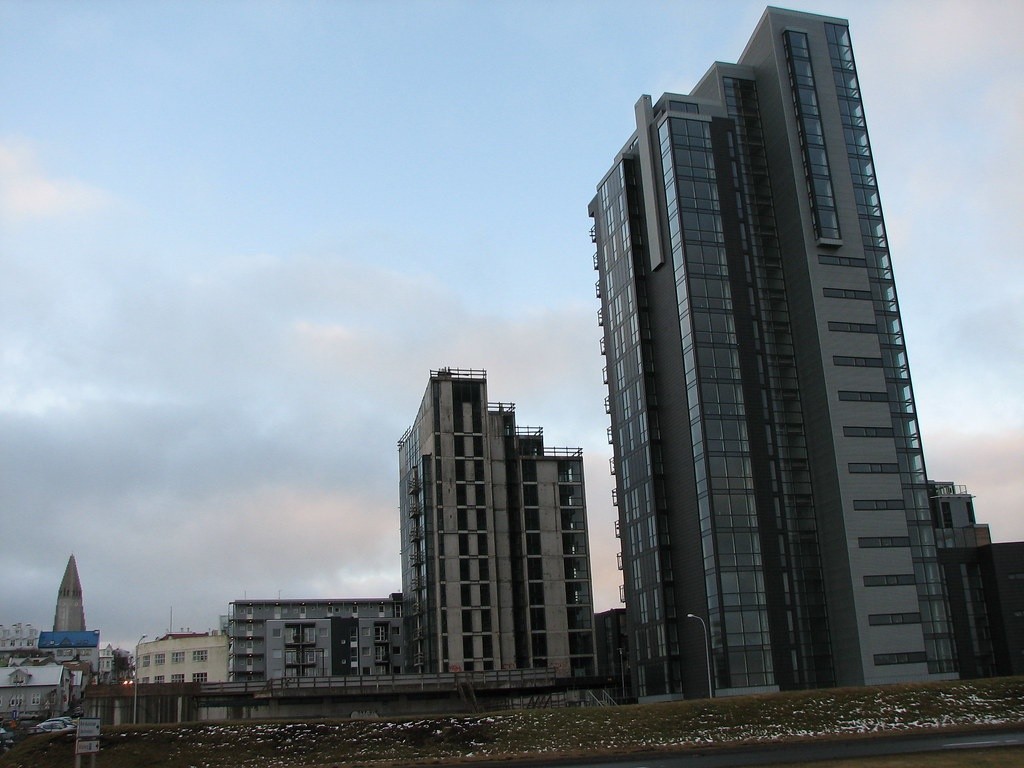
[
  {"left": 72, "top": 706, "right": 85, "bottom": 718},
  {"left": 0, "top": 716, "right": 77, "bottom": 755}
]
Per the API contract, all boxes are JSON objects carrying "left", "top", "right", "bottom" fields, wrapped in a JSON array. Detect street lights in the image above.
[
  {"left": 687, "top": 613, "right": 713, "bottom": 699},
  {"left": 133, "top": 634, "right": 149, "bottom": 724}
]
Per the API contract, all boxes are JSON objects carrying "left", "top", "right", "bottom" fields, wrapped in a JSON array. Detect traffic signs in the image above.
[
  {"left": 75, "top": 718, "right": 101, "bottom": 737},
  {"left": 75, "top": 738, "right": 100, "bottom": 754}
]
